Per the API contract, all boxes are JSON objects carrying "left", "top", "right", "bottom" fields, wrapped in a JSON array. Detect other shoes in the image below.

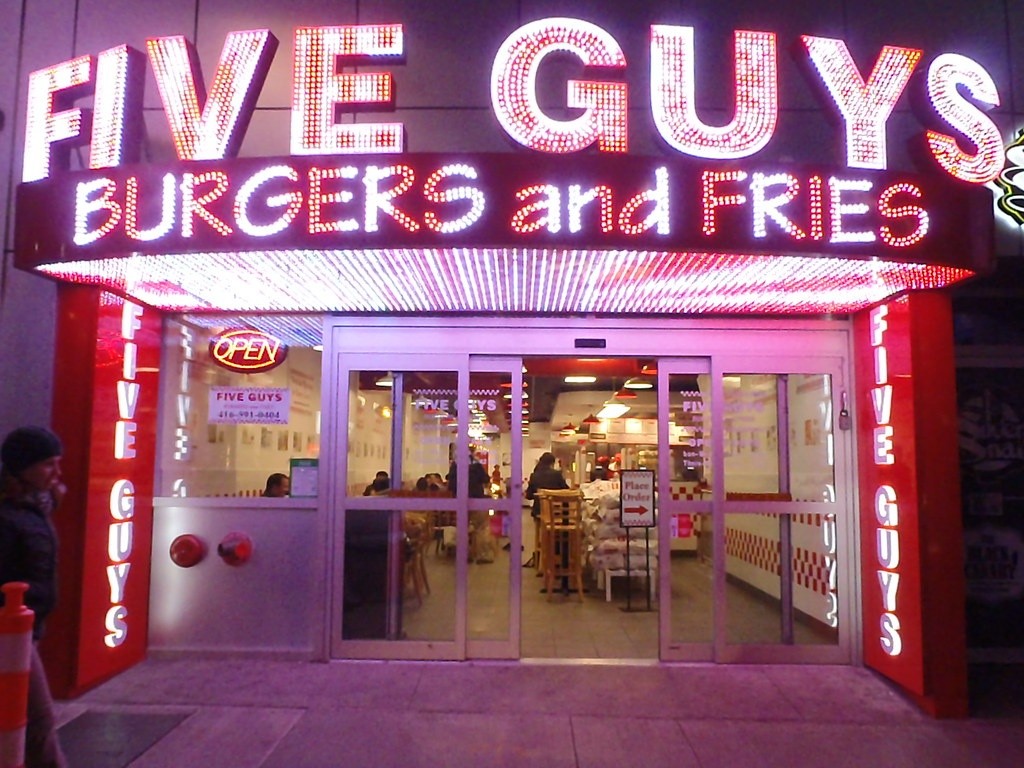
[{"left": 452, "top": 553, "right": 493, "bottom": 566}]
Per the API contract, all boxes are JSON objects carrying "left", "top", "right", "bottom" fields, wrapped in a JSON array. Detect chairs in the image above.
[
  {"left": 546, "top": 495, "right": 587, "bottom": 602},
  {"left": 403, "top": 510, "right": 435, "bottom": 606}
]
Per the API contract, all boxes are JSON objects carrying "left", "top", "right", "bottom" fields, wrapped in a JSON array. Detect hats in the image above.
[{"left": 0, "top": 426, "right": 62, "bottom": 476}]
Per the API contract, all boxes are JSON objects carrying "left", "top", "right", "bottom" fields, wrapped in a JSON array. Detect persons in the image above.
[
  {"left": 0, "top": 426, "right": 66, "bottom": 768},
  {"left": 364, "top": 446, "right": 571, "bottom": 566},
  {"left": 261, "top": 473, "right": 290, "bottom": 498}
]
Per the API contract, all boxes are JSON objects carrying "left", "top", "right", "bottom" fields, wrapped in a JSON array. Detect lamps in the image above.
[
  {"left": 411, "top": 395, "right": 458, "bottom": 434},
  {"left": 561, "top": 422, "right": 576, "bottom": 435},
  {"left": 562, "top": 374, "right": 600, "bottom": 385},
  {"left": 614, "top": 386, "right": 638, "bottom": 398},
  {"left": 563, "top": 414, "right": 580, "bottom": 431},
  {"left": 582, "top": 404, "right": 601, "bottom": 425},
  {"left": 624, "top": 377, "right": 653, "bottom": 389},
  {"left": 603, "top": 376, "right": 625, "bottom": 406},
  {"left": 642, "top": 362, "right": 658, "bottom": 374},
  {"left": 376, "top": 372, "right": 392, "bottom": 386},
  {"left": 500, "top": 364, "right": 530, "bottom": 437}
]
